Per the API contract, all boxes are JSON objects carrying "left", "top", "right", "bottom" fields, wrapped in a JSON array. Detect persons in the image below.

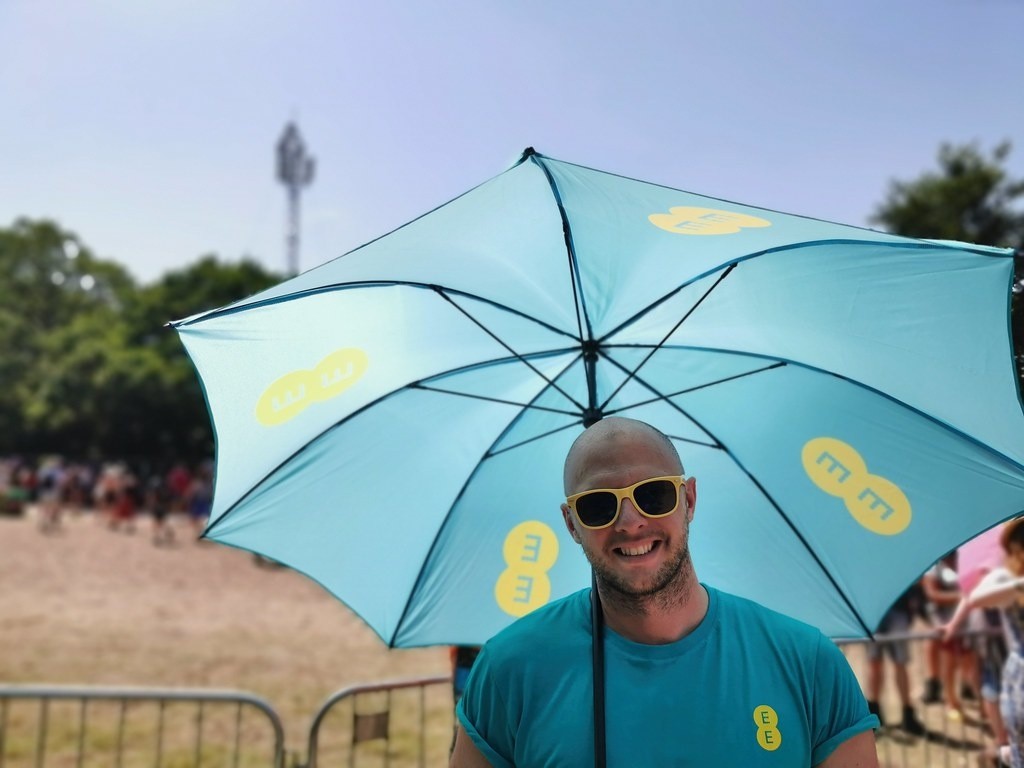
[
  {"left": 856, "top": 514, "right": 1024, "bottom": 768},
  {"left": 443, "top": 415, "right": 880, "bottom": 768}
]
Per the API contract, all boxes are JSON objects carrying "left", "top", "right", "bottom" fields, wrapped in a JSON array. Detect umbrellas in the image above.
[{"left": 164, "top": 143, "right": 1024, "bottom": 767}]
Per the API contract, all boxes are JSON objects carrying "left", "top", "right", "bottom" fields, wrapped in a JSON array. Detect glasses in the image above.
[{"left": 567, "top": 475, "right": 687, "bottom": 530}]
